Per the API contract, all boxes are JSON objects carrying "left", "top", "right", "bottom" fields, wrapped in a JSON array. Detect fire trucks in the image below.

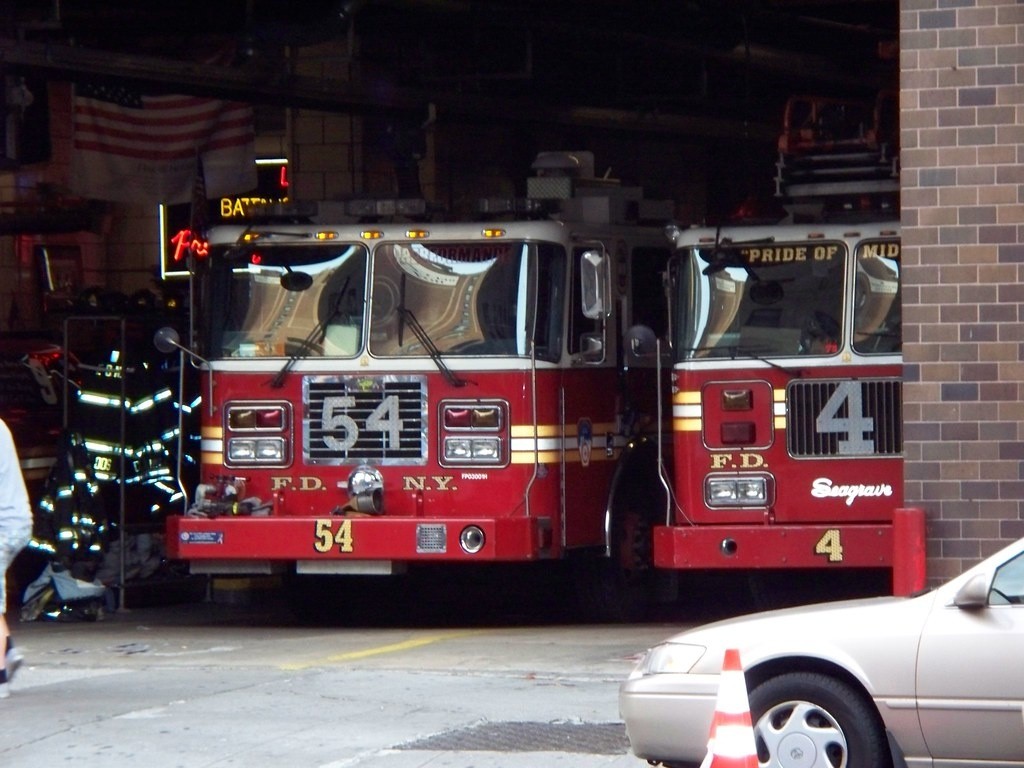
[
  {"left": 167, "top": 149, "right": 675, "bottom": 612},
  {"left": 622, "top": 39, "right": 902, "bottom": 607}
]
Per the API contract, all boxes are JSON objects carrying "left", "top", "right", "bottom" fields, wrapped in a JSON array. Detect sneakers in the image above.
[
  {"left": 0, "top": 682, "right": 11, "bottom": 700},
  {"left": 4, "top": 648, "right": 23, "bottom": 680}
]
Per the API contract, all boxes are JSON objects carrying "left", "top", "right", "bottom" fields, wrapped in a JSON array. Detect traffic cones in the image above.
[{"left": 699, "top": 649, "right": 760, "bottom": 768}]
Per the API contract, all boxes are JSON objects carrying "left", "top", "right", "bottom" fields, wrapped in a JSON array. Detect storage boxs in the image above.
[{"left": 111, "top": 577, "right": 207, "bottom": 611}]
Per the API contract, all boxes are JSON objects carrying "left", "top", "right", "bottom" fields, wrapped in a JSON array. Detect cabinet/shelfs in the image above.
[{"left": 61, "top": 313, "right": 216, "bottom": 616}]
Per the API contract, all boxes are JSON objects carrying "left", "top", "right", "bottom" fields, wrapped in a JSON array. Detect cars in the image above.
[{"left": 617, "top": 538, "right": 1024, "bottom": 768}]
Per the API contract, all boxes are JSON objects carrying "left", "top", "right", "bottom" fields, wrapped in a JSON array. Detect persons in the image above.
[{"left": 0, "top": 417, "right": 34, "bottom": 693}]
